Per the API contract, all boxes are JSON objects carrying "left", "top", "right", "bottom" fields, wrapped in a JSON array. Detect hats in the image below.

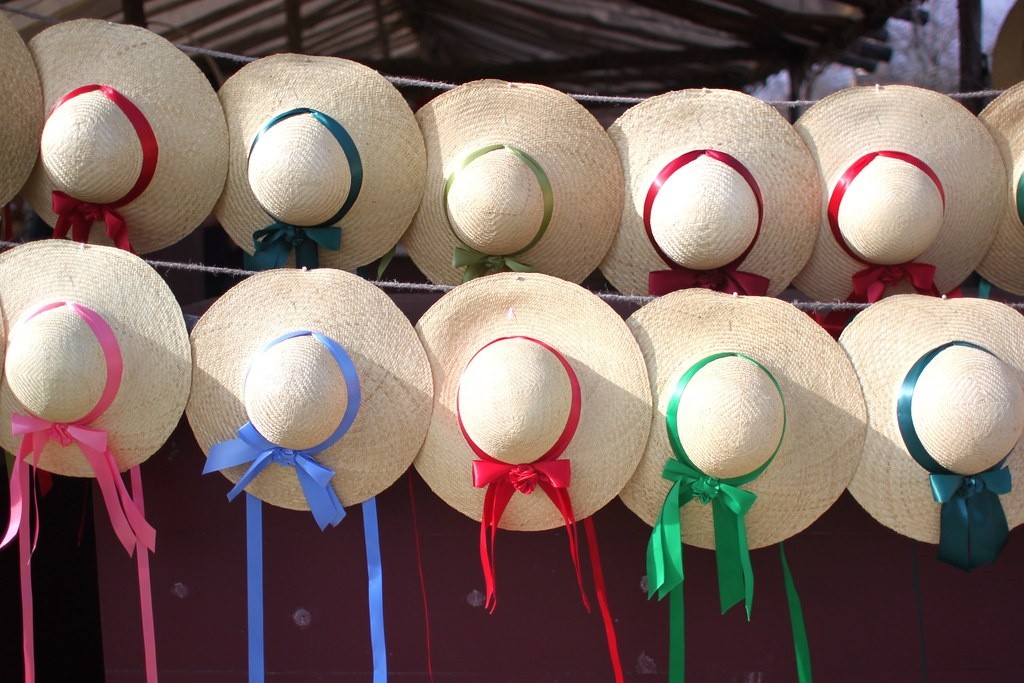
[
  {"left": 0, "top": 12, "right": 44, "bottom": 206},
  {"left": 213, "top": 52, "right": 427, "bottom": 272},
  {"left": 411, "top": 270, "right": 653, "bottom": 683},
  {"left": 0, "top": 238, "right": 193, "bottom": 683},
  {"left": 21, "top": 18, "right": 229, "bottom": 254},
  {"left": 596, "top": 86, "right": 821, "bottom": 304},
  {"left": 404, "top": 78, "right": 625, "bottom": 290},
  {"left": 790, "top": 84, "right": 1008, "bottom": 306},
  {"left": 186, "top": 266, "right": 435, "bottom": 683},
  {"left": 619, "top": 287, "right": 867, "bottom": 683},
  {"left": 975, "top": 82, "right": 1024, "bottom": 297},
  {"left": 837, "top": 293, "right": 1024, "bottom": 572}
]
